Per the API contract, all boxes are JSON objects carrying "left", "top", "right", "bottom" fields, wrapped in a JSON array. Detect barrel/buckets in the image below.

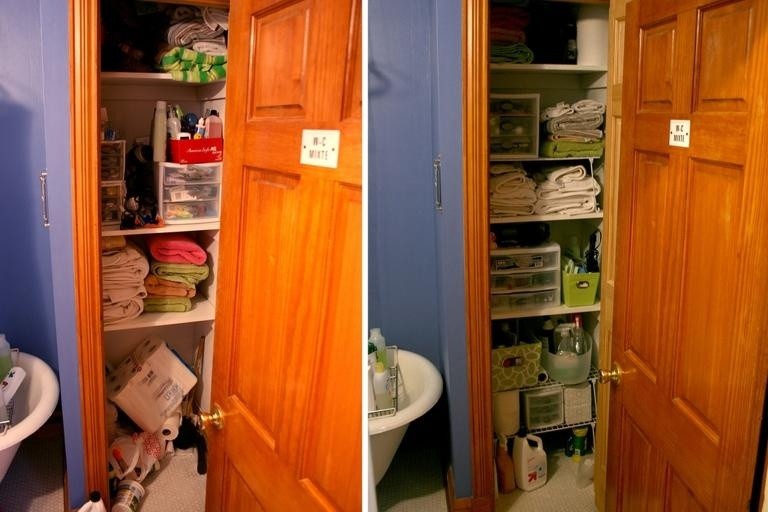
[
  {"left": 512, "top": 428, "right": 548, "bottom": 491},
  {"left": 79, "top": 493, "right": 107, "bottom": 512}
]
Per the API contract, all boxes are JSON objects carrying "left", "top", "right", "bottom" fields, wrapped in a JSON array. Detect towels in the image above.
[
  {"left": 101, "top": 233, "right": 210, "bottom": 326},
  {"left": 489, "top": 164, "right": 601, "bottom": 216},
  {"left": 542, "top": 97, "right": 606, "bottom": 159}
]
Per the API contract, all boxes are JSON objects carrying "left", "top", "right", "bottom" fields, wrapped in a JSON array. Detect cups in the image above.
[{"left": 111, "top": 479, "right": 145, "bottom": 512}]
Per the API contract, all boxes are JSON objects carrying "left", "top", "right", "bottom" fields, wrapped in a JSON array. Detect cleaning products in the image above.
[{"left": 497, "top": 433, "right": 516, "bottom": 494}]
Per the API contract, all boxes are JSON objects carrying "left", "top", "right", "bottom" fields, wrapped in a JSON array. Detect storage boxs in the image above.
[
  {"left": 491, "top": 336, "right": 543, "bottom": 392},
  {"left": 561, "top": 268, "right": 601, "bottom": 307},
  {"left": 488, "top": 93, "right": 542, "bottom": 159},
  {"left": 527, "top": 389, "right": 564, "bottom": 429},
  {"left": 544, "top": 332, "right": 593, "bottom": 386},
  {"left": 167, "top": 138, "right": 223, "bottom": 164},
  {"left": 101, "top": 140, "right": 126, "bottom": 183},
  {"left": 489, "top": 244, "right": 561, "bottom": 314},
  {"left": 156, "top": 160, "right": 221, "bottom": 224},
  {"left": 102, "top": 184, "right": 123, "bottom": 226}
]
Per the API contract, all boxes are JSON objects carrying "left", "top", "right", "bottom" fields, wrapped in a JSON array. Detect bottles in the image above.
[
  {"left": 542, "top": 321, "right": 556, "bottom": 351},
  {"left": 574, "top": 316, "right": 587, "bottom": 353}
]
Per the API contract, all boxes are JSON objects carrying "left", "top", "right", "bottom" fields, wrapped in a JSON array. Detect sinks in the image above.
[
  {"left": 368, "top": 349, "right": 443, "bottom": 488},
  {"left": 0, "top": 353, "right": 59, "bottom": 484}
]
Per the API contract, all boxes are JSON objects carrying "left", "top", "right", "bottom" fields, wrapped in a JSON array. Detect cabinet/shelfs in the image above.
[
  {"left": 489, "top": 61, "right": 607, "bottom": 442},
  {"left": 102, "top": 1, "right": 230, "bottom": 331}
]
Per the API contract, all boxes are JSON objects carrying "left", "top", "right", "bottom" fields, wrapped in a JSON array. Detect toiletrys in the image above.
[{"left": 151, "top": 100, "right": 222, "bottom": 163}]
